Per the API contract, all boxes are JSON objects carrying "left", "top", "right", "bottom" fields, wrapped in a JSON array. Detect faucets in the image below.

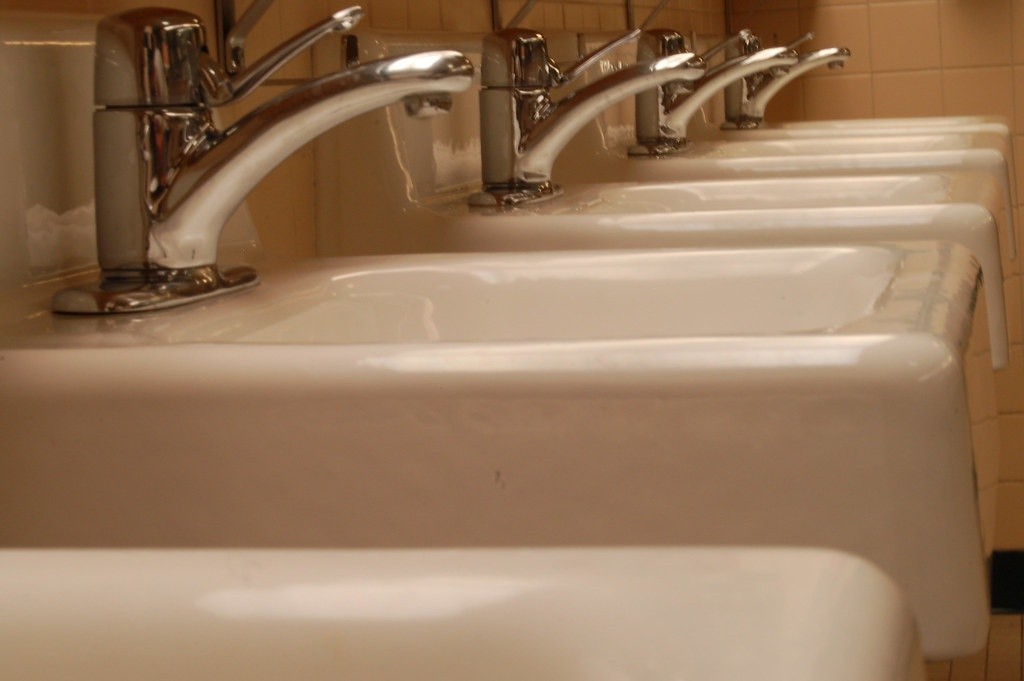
[
  {"left": 717, "top": 29, "right": 853, "bottom": 131},
  {"left": 625, "top": 28, "right": 799, "bottom": 155},
  {"left": 463, "top": 28, "right": 709, "bottom": 207},
  {"left": 48, "top": 6, "right": 475, "bottom": 319}
]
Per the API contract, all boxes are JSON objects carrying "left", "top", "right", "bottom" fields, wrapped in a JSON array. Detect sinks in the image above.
[
  {"left": 1, "top": 241, "right": 1005, "bottom": 680},
  {"left": 616, "top": 113, "right": 1019, "bottom": 264},
  {"left": 553, "top": 173, "right": 1011, "bottom": 371}
]
